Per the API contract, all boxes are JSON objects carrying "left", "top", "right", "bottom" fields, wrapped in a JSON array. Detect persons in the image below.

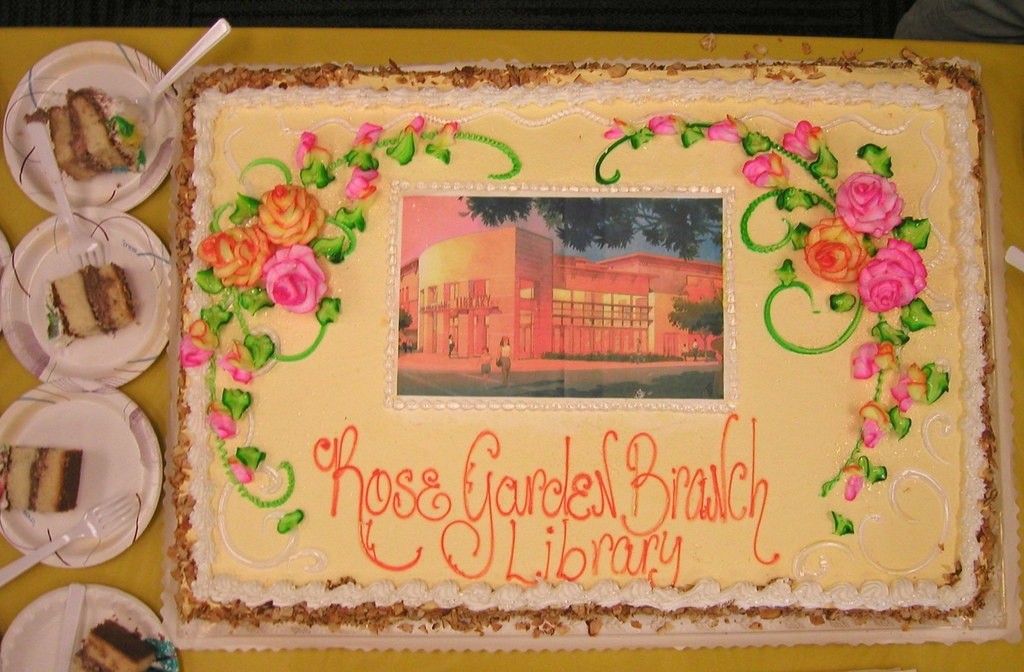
[{"left": 893, "top": 0, "right": 1024, "bottom": 46}]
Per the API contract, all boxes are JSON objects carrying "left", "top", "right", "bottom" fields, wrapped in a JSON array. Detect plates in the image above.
[
  {"left": 0, "top": 377, "right": 164, "bottom": 569},
  {"left": 1, "top": 206, "right": 180, "bottom": 388},
  {"left": 2, "top": 40, "right": 183, "bottom": 215},
  {"left": 0, "top": 582, "right": 181, "bottom": 672}
]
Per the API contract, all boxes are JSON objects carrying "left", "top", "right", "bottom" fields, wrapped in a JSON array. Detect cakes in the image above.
[
  {"left": 47, "top": 87, "right": 150, "bottom": 183},
  {"left": 69, "top": 618, "right": 157, "bottom": 672},
  {"left": 161, "top": 46, "right": 1000, "bottom": 636},
  {"left": 45, "top": 262, "right": 136, "bottom": 339},
  {"left": 0, "top": 444, "right": 84, "bottom": 513}
]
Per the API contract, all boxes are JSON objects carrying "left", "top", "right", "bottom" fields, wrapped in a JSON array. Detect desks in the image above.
[{"left": 0, "top": 30, "right": 1024, "bottom": 672}]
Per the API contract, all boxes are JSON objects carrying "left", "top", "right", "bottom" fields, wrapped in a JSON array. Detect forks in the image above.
[
  {"left": 0, "top": 489, "right": 135, "bottom": 589},
  {"left": 25, "top": 120, "right": 105, "bottom": 270},
  {"left": 138, "top": 17, "right": 232, "bottom": 126}
]
